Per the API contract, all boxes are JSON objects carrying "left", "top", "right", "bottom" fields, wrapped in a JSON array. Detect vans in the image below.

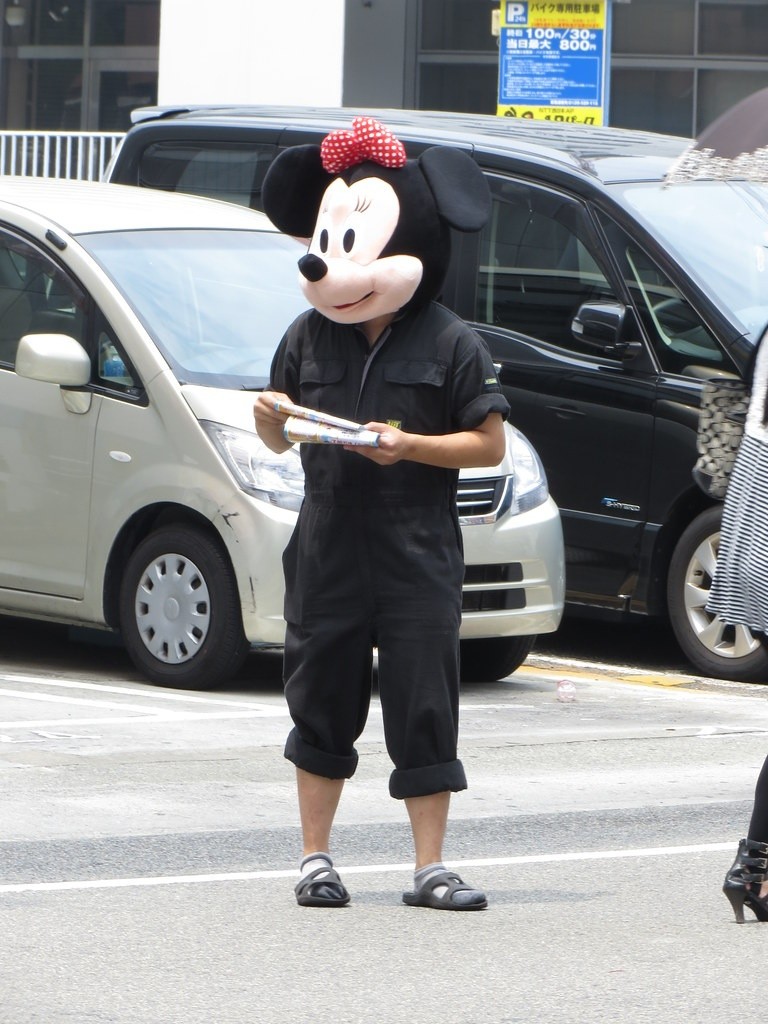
[
  {"left": 0, "top": 175, "right": 568, "bottom": 691},
  {"left": 78, "top": 101, "right": 768, "bottom": 684}
]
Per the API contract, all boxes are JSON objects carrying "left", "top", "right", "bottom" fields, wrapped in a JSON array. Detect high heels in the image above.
[{"left": 722, "top": 839, "right": 768, "bottom": 923}]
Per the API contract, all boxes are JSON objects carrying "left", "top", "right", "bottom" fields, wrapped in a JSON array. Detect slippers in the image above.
[
  {"left": 295, "top": 867, "right": 350, "bottom": 906},
  {"left": 402, "top": 873, "right": 488, "bottom": 910}
]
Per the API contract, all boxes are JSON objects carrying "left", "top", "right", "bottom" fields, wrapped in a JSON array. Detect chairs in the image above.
[{"left": 0, "top": 288, "right": 34, "bottom": 363}]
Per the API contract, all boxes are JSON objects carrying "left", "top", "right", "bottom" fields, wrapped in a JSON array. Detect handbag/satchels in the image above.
[{"left": 691, "top": 377, "right": 753, "bottom": 501}]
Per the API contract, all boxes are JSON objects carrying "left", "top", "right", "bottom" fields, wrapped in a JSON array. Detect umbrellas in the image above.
[{"left": 660, "top": 86, "right": 768, "bottom": 186}]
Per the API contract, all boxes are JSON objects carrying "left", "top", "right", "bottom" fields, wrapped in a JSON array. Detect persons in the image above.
[
  {"left": 254, "top": 119, "right": 510, "bottom": 912},
  {"left": 704, "top": 323, "right": 767, "bottom": 925}
]
